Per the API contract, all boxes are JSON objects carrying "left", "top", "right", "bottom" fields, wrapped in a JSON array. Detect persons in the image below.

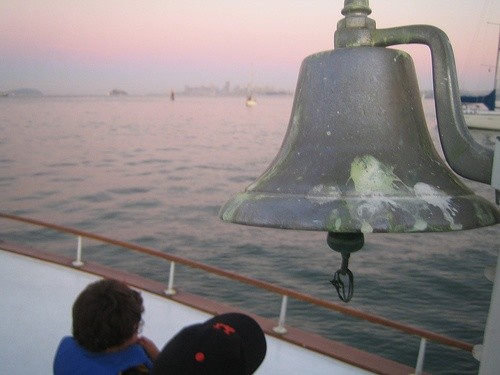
[
  {"left": 54, "top": 279, "right": 161, "bottom": 375},
  {"left": 154, "top": 312, "right": 268, "bottom": 375}
]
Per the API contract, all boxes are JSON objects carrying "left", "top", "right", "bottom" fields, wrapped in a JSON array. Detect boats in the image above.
[{"left": 246, "top": 96, "right": 257, "bottom": 106}]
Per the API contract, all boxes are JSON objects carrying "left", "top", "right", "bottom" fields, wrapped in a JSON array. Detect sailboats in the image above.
[{"left": 460, "top": 21, "right": 500, "bottom": 134}]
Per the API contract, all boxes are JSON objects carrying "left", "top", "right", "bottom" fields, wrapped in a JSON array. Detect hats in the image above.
[{"left": 152, "top": 312, "right": 267, "bottom": 374}]
